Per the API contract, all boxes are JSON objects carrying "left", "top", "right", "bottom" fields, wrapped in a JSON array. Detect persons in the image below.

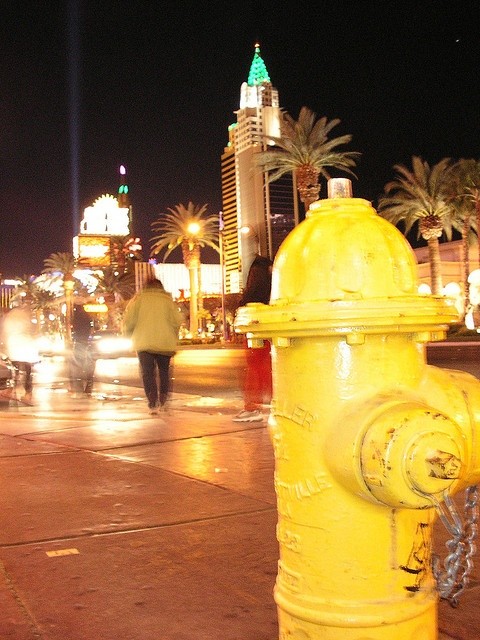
[
  {"left": 121, "top": 276, "right": 183, "bottom": 408},
  {"left": 70, "top": 298, "right": 93, "bottom": 396},
  {"left": 232, "top": 253, "right": 273, "bottom": 423}
]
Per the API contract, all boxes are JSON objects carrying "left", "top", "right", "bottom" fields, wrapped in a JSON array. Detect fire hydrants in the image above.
[{"left": 234, "top": 177, "right": 479, "bottom": 639}]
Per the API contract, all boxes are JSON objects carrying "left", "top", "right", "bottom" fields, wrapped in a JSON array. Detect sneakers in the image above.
[{"left": 231, "top": 408, "right": 264, "bottom": 422}]
